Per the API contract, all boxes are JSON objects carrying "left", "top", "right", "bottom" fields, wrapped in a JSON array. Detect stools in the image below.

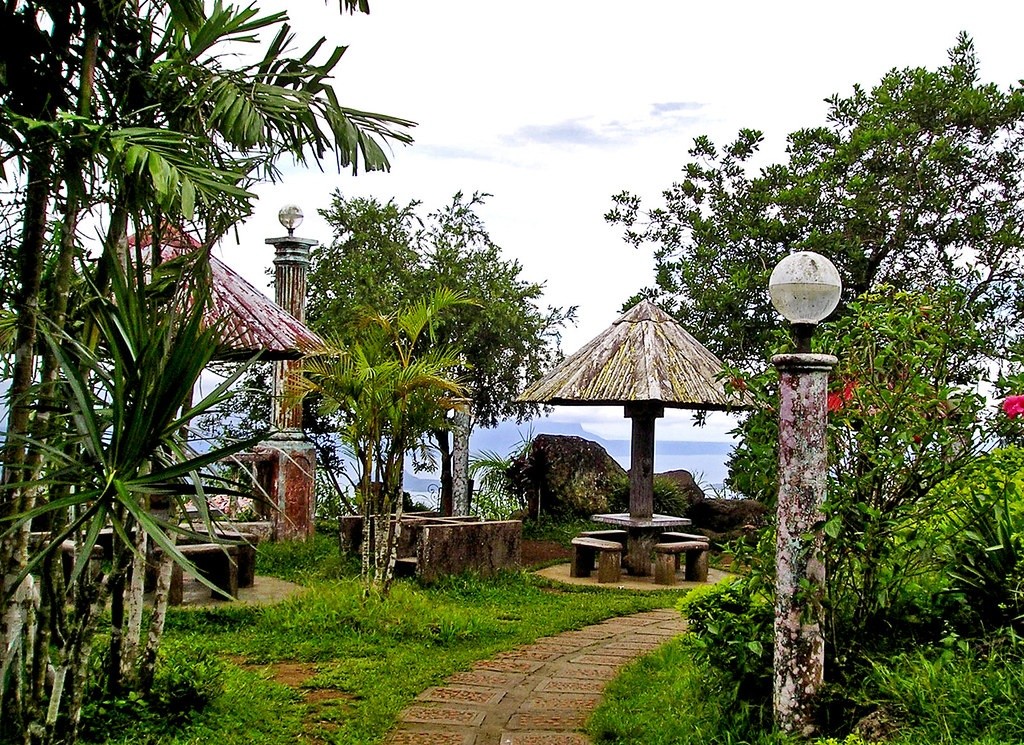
[
  {"left": 577, "top": 529, "right": 628, "bottom": 559},
  {"left": 570, "top": 536, "right": 623, "bottom": 583},
  {"left": 76, "top": 525, "right": 139, "bottom": 561},
  {"left": 652, "top": 539, "right": 710, "bottom": 585},
  {"left": 41, "top": 538, "right": 103, "bottom": 598},
  {"left": 661, "top": 530, "right": 710, "bottom": 570},
  {"left": 151, "top": 542, "right": 239, "bottom": 606},
  {"left": 195, "top": 528, "right": 259, "bottom": 588}
]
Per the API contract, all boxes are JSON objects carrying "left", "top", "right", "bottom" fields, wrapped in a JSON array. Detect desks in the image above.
[{"left": 591, "top": 512, "right": 692, "bottom": 577}]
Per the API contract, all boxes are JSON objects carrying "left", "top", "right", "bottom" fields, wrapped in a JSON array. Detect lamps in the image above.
[
  {"left": 278, "top": 204, "right": 305, "bottom": 237},
  {"left": 768, "top": 250, "right": 843, "bottom": 352}
]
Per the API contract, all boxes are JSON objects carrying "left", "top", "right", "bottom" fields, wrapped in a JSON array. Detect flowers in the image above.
[{"left": 1003, "top": 393, "right": 1024, "bottom": 419}]
